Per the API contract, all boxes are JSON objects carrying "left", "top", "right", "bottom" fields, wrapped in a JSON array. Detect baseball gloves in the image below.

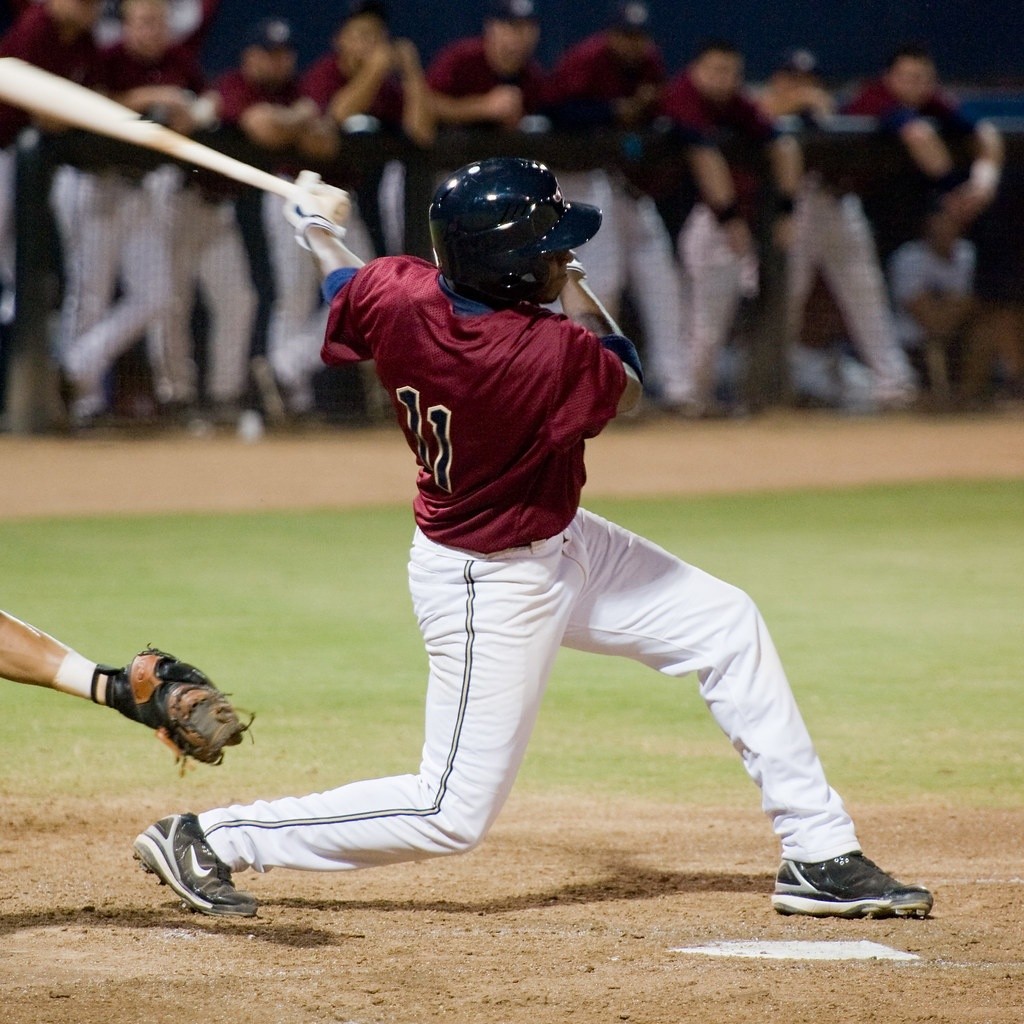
[{"left": 89, "top": 647, "right": 243, "bottom": 767}]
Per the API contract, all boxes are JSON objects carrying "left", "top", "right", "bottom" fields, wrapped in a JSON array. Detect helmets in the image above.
[{"left": 428, "top": 158, "right": 602, "bottom": 299}]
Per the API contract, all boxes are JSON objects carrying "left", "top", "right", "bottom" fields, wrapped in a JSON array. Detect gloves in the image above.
[
  {"left": 566, "top": 250, "right": 587, "bottom": 279},
  {"left": 281, "top": 169, "right": 353, "bottom": 251}
]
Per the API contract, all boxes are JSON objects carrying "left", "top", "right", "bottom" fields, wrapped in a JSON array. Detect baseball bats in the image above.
[{"left": 0, "top": 56, "right": 353, "bottom": 228}]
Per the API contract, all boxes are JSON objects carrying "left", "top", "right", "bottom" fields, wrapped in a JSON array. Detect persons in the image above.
[
  {"left": 133, "top": 159, "right": 940, "bottom": 917},
  {"left": 0, "top": 610, "right": 258, "bottom": 777},
  {"left": 0, "top": 0, "right": 1024, "bottom": 432}
]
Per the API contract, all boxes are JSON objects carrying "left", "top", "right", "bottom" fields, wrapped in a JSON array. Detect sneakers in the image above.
[
  {"left": 773, "top": 851, "right": 933, "bottom": 919},
  {"left": 131, "top": 811, "right": 258, "bottom": 916}
]
